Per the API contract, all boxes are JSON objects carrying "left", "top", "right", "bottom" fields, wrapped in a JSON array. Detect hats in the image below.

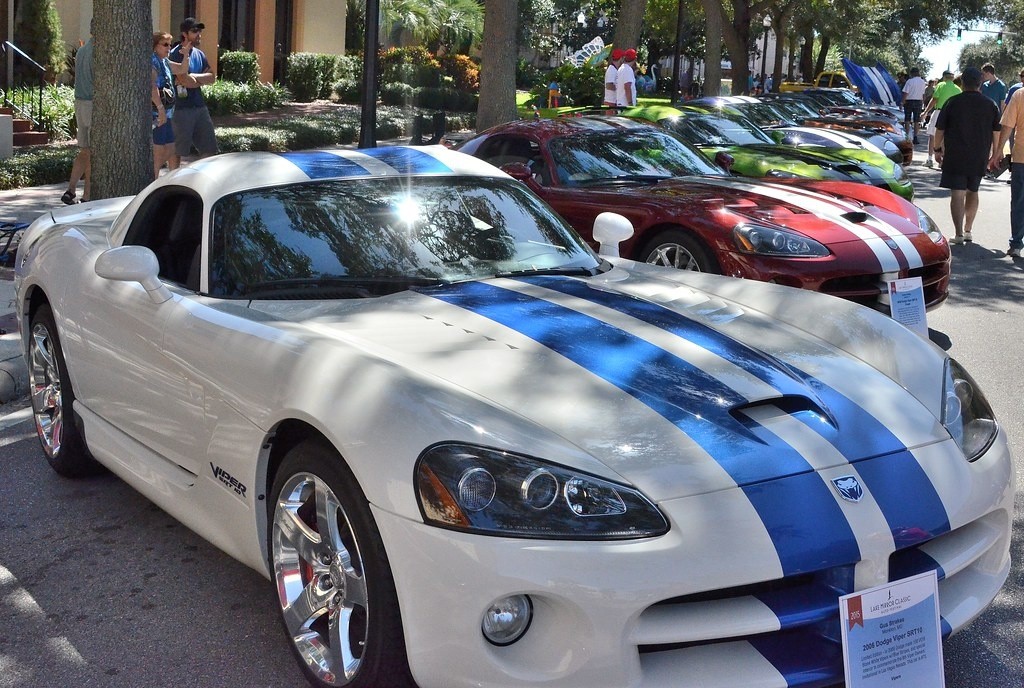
[
  {"left": 625, "top": 48, "right": 637, "bottom": 61},
  {"left": 180, "top": 18, "right": 204, "bottom": 32},
  {"left": 943, "top": 71, "right": 950, "bottom": 76},
  {"left": 911, "top": 68, "right": 918, "bottom": 73},
  {"left": 612, "top": 49, "right": 625, "bottom": 61}
]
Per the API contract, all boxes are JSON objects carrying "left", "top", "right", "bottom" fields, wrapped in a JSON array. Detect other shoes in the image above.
[
  {"left": 923, "top": 160, "right": 933, "bottom": 166},
  {"left": 913, "top": 138, "right": 919, "bottom": 144},
  {"left": 1007, "top": 174, "right": 1012, "bottom": 183},
  {"left": 938, "top": 161, "right": 943, "bottom": 168},
  {"left": 983, "top": 170, "right": 994, "bottom": 179}
]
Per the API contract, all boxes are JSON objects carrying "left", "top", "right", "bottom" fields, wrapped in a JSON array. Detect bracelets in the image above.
[
  {"left": 934, "top": 147, "right": 941, "bottom": 152},
  {"left": 157, "top": 103, "right": 163, "bottom": 110},
  {"left": 628, "top": 102, "right": 632, "bottom": 104}
]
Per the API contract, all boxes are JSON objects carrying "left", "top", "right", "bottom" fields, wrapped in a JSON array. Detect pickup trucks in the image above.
[{"left": 779, "top": 68, "right": 862, "bottom": 99}]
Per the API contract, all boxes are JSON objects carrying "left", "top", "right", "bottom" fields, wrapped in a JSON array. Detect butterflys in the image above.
[{"left": 567, "top": 35, "right": 614, "bottom": 70}]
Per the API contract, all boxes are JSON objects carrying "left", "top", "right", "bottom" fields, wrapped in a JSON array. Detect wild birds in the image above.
[{"left": 635, "top": 63, "right": 658, "bottom": 93}]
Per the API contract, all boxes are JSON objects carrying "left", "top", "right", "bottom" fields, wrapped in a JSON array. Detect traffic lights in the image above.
[
  {"left": 957, "top": 27, "right": 962, "bottom": 41},
  {"left": 996, "top": 33, "right": 1003, "bottom": 45}
]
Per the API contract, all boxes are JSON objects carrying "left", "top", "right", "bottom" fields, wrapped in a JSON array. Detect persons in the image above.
[
  {"left": 898, "top": 67, "right": 939, "bottom": 145},
  {"left": 152, "top": 31, "right": 192, "bottom": 180},
  {"left": 169, "top": 17, "right": 221, "bottom": 163},
  {"left": 933, "top": 66, "right": 1002, "bottom": 243},
  {"left": 981, "top": 63, "right": 1007, "bottom": 114},
  {"left": 681, "top": 68, "right": 692, "bottom": 96},
  {"left": 636, "top": 71, "right": 645, "bottom": 86},
  {"left": 782, "top": 75, "right": 787, "bottom": 82},
  {"left": 993, "top": 67, "right": 1024, "bottom": 257},
  {"left": 749, "top": 71, "right": 773, "bottom": 95},
  {"left": 61, "top": 19, "right": 94, "bottom": 205},
  {"left": 796, "top": 75, "right": 803, "bottom": 82},
  {"left": 604, "top": 48, "right": 637, "bottom": 114},
  {"left": 920, "top": 70, "right": 963, "bottom": 169}
]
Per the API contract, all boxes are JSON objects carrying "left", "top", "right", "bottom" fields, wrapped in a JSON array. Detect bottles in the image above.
[{"left": 177, "top": 82, "right": 188, "bottom": 97}]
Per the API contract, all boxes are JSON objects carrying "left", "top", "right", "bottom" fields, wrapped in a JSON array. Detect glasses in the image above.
[
  {"left": 157, "top": 42, "right": 173, "bottom": 48},
  {"left": 1020, "top": 75, "right": 1024, "bottom": 78},
  {"left": 189, "top": 27, "right": 202, "bottom": 33},
  {"left": 899, "top": 75, "right": 903, "bottom": 78}
]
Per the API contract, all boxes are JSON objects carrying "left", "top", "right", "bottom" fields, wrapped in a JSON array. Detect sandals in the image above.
[{"left": 61, "top": 190, "right": 89, "bottom": 205}]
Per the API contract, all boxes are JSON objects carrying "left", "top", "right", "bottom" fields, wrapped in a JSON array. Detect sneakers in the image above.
[
  {"left": 1007, "top": 246, "right": 1020, "bottom": 257},
  {"left": 949, "top": 235, "right": 964, "bottom": 244},
  {"left": 963, "top": 231, "right": 972, "bottom": 241}
]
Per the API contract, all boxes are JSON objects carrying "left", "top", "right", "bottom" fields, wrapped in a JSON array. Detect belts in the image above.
[{"left": 934, "top": 107, "right": 942, "bottom": 110}]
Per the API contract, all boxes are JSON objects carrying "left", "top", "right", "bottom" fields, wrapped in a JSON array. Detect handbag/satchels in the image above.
[{"left": 149, "top": 55, "right": 175, "bottom": 107}]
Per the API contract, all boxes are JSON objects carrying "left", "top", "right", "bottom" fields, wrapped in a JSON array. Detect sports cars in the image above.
[
  {"left": 439, "top": 109, "right": 952, "bottom": 318},
  {"left": 535, "top": 56, "right": 917, "bottom": 205},
  {"left": 13, "top": 140, "right": 1016, "bottom": 688}
]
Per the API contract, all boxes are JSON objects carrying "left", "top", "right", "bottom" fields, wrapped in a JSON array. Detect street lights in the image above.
[{"left": 760, "top": 15, "right": 771, "bottom": 94}]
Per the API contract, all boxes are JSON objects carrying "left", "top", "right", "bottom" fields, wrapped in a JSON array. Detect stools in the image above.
[{"left": 0, "top": 221, "right": 31, "bottom": 266}]
